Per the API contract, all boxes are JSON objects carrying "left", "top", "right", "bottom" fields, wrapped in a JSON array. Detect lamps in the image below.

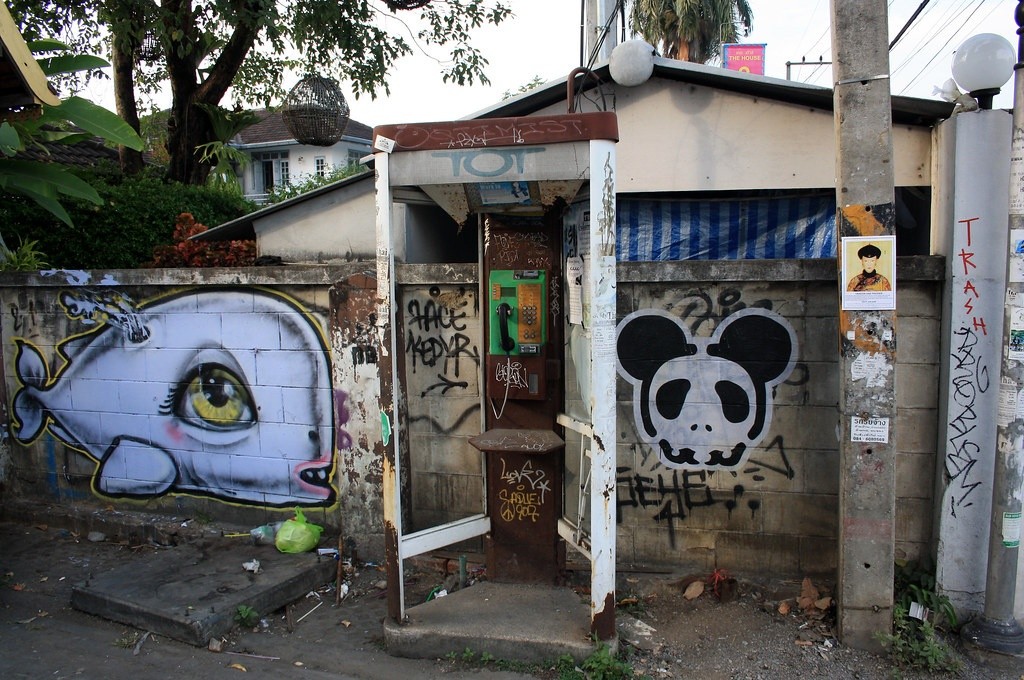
[
  {"left": 298, "top": 156, "right": 304, "bottom": 165},
  {"left": 952, "top": 33, "right": 1017, "bottom": 108}
]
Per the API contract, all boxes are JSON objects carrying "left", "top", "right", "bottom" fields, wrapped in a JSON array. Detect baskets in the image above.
[{"left": 281, "top": 78, "right": 350, "bottom": 147}]
